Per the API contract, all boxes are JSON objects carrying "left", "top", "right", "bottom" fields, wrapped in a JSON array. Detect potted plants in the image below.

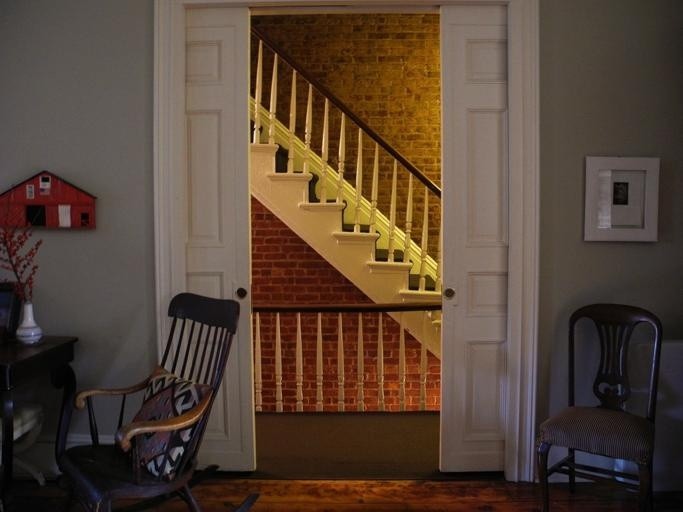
[{"left": 1, "top": 189, "right": 43, "bottom": 306}]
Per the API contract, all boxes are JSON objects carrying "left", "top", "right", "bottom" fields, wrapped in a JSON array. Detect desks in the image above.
[{"left": 0, "top": 327, "right": 79, "bottom": 511}]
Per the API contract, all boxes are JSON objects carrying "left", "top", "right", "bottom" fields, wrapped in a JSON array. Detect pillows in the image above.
[{"left": 114, "top": 364, "right": 213, "bottom": 484}]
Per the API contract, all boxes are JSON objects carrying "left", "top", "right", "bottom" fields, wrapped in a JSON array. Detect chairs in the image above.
[
  {"left": 58, "top": 292, "right": 261, "bottom": 512},
  {"left": 538, "top": 302, "right": 664, "bottom": 512}
]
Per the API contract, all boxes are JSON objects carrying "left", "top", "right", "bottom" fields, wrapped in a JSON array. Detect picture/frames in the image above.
[{"left": 582, "top": 153, "right": 661, "bottom": 245}]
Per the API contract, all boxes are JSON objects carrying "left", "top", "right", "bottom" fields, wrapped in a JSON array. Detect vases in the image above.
[{"left": 14, "top": 301, "right": 43, "bottom": 347}]
